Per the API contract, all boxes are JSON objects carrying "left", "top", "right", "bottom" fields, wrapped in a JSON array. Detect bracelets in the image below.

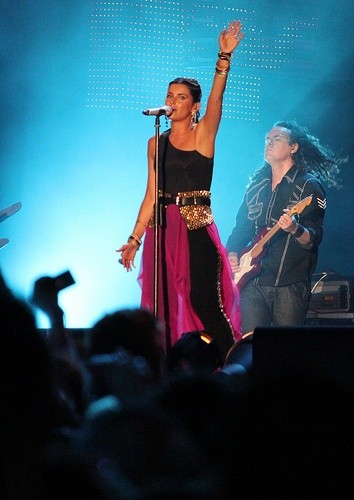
[
  {"left": 127, "top": 233, "right": 141, "bottom": 250},
  {"left": 215, "top": 51, "right": 231, "bottom": 75},
  {"left": 291, "top": 224, "right": 304, "bottom": 238},
  {"left": 227, "top": 253, "right": 237, "bottom": 256}
]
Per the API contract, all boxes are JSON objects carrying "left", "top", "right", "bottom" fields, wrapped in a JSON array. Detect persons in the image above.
[
  {"left": 224, "top": 119, "right": 348, "bottom": 334},
  {"left": 114, "top": 18, "right": 242, "bottom": 369},
  {"left": 0, "top": 267, "right": 258, "bottom": 500}
]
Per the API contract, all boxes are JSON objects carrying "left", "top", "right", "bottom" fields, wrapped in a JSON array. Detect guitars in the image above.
[{"left": 231, "top": 192, "right": 315, "bottom": 295}]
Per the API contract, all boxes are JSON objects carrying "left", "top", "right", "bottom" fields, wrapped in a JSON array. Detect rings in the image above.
[
  {"left": 281, "top": 222, "right": 284, "bottom": 225},
  {"left": 118, "top": 259, "right": 127, "bottom": 265},
  {"left": 232, "top": 34, "right": 237, "bottom": 37}
]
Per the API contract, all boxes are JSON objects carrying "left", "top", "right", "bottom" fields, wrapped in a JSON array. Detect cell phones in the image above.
[{"left": 53, "top": 271, "right": 75, "bottom": 291}]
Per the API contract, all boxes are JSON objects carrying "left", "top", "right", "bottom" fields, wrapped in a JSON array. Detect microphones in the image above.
[{"left": 143, "top": 105, "right": 173, "bottom": 116}]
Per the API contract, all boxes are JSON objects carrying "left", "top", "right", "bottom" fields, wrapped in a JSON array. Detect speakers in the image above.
[{"left": 250, "top": 323, "right": 354, "bottom": 394}]
